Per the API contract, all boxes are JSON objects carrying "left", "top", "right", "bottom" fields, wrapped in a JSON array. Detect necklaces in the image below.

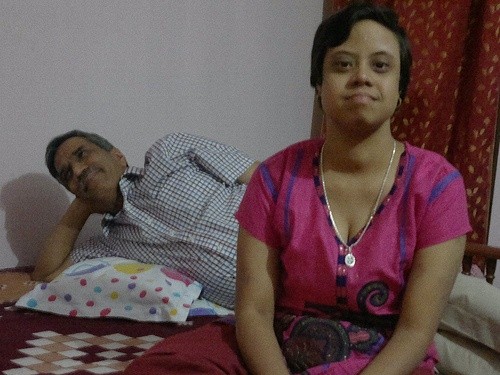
[{"left": 320, "top": 138, "right": 397, "bottom": 269}]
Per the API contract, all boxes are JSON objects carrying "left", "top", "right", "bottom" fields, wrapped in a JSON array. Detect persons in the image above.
[
  {"left": 29, "top": 128, "right": 499, "bottom": 374},
  {"left": 122, "top": 4, "right": 471, "bottom": 374}
]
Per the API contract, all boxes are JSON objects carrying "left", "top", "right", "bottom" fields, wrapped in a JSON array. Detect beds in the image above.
[{"left": 0, "top": 263, "right": 225, "bottom": 375}]
